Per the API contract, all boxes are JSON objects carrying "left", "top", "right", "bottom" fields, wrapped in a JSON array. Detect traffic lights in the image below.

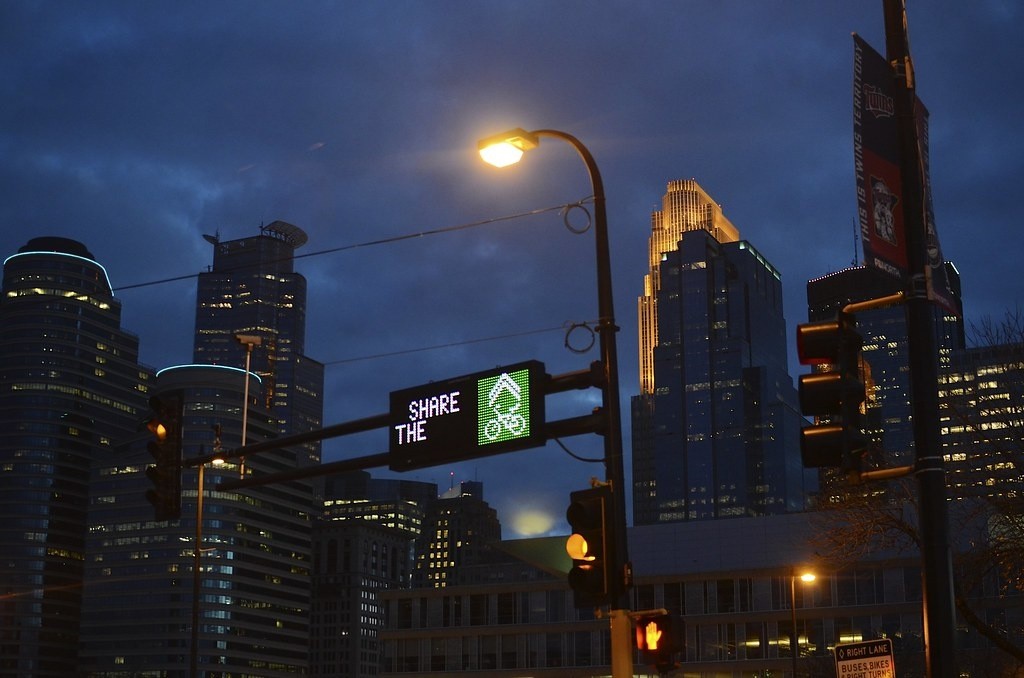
[
  {"left": 635, "top": 614, "right": 669, "bottom": 663},
  {"left": 566, "top": 487, "right": 616, "bottom": 609},
  {"left": 148, "top": 404, "right": 187, "bottom": 523},
  {"left": 793, "top": 316, "right": 867, "bottom": 469}
]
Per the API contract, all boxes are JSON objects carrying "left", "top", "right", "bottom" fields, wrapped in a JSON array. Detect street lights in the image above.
[
  {"left": 789, "top": 571, "right": 816, "bottom": 678},
  {"left": 186, "top": 455, "right": 225, "bottom": 678},
  {"left": 475, "top": 125, "right": 634, "bottom": 678}
]
